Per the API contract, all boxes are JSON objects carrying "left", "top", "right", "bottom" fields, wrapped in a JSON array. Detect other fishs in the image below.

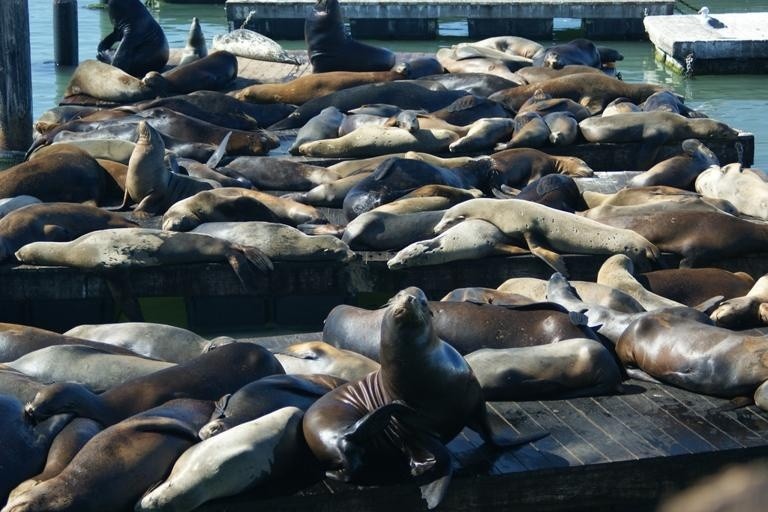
[{"left": 0, "top": 0, "right": 767, "bottom": 512}]
